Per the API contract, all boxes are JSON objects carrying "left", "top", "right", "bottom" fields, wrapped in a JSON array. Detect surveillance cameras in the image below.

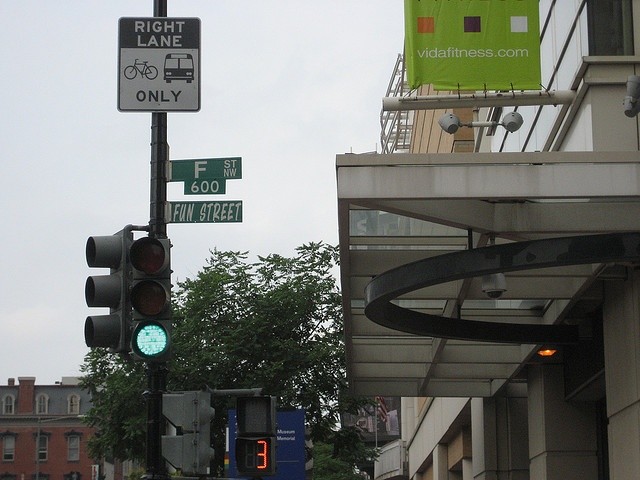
[{"left": 482, "top": 232, "right": 508, "bottom": 299}]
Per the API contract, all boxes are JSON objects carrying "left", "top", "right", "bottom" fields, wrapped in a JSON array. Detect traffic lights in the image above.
[
  {"left": 162, "top": 392, "right": 196, "bottom": 474},
  {"left": 195, "top": 391, "right": 215, "bottom": 476},
  {"left": 130, "top": 237, "right": 174, "bottom": 363},
  {"left": 236, "top": 395, "right": 276, "bottom": 475},
  {"left": 84, "top": 229, "right": 133, "bottom": 352}
]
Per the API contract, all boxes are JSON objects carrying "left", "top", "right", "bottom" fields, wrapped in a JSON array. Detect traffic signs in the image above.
[
  {"left": 167, "top": 200, "right": 242, "bottom": 223},
  {"left": 118, "top": 16, "right": 201, "bottom": 113},
  {"left": 166, "top": 157, "right": 242, "bottom": 195}
]
